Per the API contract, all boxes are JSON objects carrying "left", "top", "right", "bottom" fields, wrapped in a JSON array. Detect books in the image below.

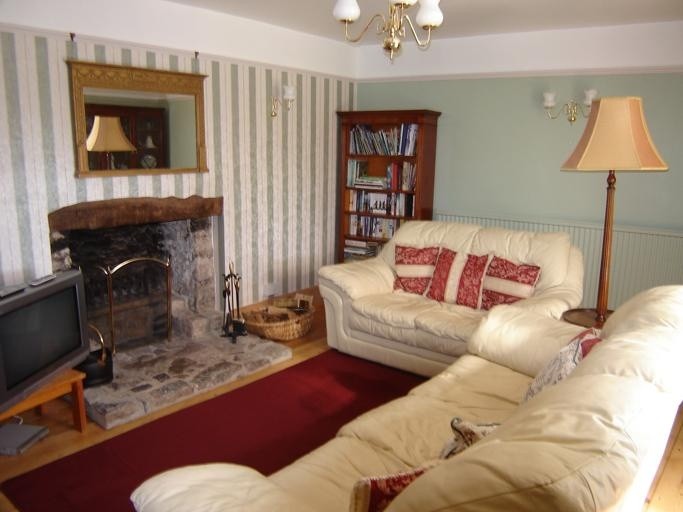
[
  {"left": 347, "top": 159, "right": 417, "bottom": 191},
  {"left": 350, "top": 124, "right": 417, "bottom": 156},
  {"left": 343, "top": 240, "right": 386, "bottom": 261},
  {"left": 346, "top": 189, "right": 415, "bottom": 217},
  {"left": 345, "top": 215, "right": 404, "bottom": 239}
]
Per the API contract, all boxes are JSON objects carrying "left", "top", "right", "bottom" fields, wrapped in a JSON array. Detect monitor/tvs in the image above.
[{"left": 0, "top": 269, "right": 90, "bottom": 418}]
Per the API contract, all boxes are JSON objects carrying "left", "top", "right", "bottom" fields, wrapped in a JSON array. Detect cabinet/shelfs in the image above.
[
  {"left": 336, "top": 109, "right": 440, "bottom": 264},
  {"left": 85, "top": 103, "right": 165, "bottom": 171}
]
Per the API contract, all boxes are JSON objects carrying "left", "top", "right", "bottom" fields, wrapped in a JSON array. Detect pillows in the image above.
[
  {"left": 394, "top": 245, "right": 441, "bottom": 295},
  {"left": 482, "top": 256, "right": 541, "bottom": 311},
  {"left": 568, "top": 327, "right": 603, "bottom": 357},
  {"left": 351, "top": 462, "right": 439, "bottom": 512},
  {"left": 440, "top": 418, "right": 503, "bottom": 462},
  {"left": 525, "top": 338, "right": 582, "bottom": 402},
  {"left": 424, "top": 246, "right": 494, "bottom": 310}
]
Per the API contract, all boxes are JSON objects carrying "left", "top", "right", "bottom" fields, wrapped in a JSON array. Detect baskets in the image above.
[{"left": 230, "top": 299, "right": 313, "bottom": 340}]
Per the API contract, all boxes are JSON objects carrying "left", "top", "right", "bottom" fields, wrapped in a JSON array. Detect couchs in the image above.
[
  {"left": 316, "top": 220, "right": 584, "bottom": 377},
  {"left": 130, "top": 286, "right": 682, "bottom": 512}
]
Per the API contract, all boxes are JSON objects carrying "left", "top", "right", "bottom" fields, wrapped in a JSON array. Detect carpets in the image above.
[{"left": 0, "top": 349, "right": 431, "bottom": 512}]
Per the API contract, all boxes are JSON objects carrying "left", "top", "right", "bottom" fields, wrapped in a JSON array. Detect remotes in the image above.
[
  {"left": 28, "top": 274, "right": 56, "bottom": 286},
  {"left": 0, "top": 284, "right": 25, "bottom": 297}
]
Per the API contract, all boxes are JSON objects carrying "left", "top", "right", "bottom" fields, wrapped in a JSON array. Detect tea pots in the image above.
[{"left": 73, "top": 325, "right": 114, "bottom": 388}]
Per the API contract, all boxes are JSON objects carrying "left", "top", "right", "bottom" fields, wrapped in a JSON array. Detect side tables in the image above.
[{"left": 0, "top": 368, "right": 88, "bottom": 434}]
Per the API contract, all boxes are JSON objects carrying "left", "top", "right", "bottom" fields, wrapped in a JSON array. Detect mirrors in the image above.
[{"left": 66, "top": 59, "right": 209, "bottom": 178}]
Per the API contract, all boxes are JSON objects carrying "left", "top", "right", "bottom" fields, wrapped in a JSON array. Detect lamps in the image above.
[
  {"left": 333, "top": 0, "right": 444, "bottom": 61},
  {"left": 86, "top": 114, "right": 137, "bottom": 170},
  {"left": 271, "top": 83, "right": 296, "bottom": 118},
  {"left": 557, "top": 97, "right": 668, "bottom": 331},
  {"left": 542, "top": 88, "right": 597, "bottom": 123}
]
